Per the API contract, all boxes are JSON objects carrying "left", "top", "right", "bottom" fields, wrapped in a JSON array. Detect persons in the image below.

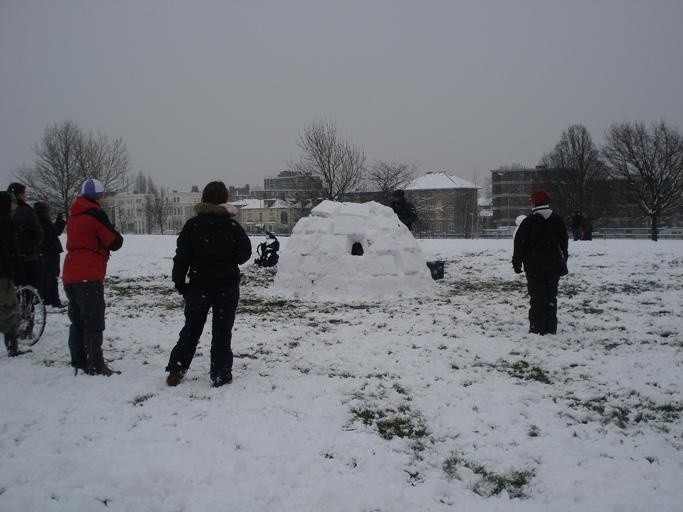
[
  {"left": 388, "top": 189, "right": 418, "bottom": 231},
  {"left": 6, "top": 181, "right": 46, "bottom": 342},
  {"left": 162, "top": 179, "right": 253, "bottom": 387},
  {"left": 31, "top": 200, "right": 68, "bottom": 312},
  {"left": 510, "top": 189, "right": 569, "bottom": 335},
  {"left": 579, "top": 222, "right": 593, "bottom": 241},
  {"left": 60, "top": 176, "right": 124, "bottom": 378},
  {"left": 0, "top": 189, "right": 35, "bottom": 359},
  {"left": 571, "top": 223, "right": 578, "bottom": 241}
]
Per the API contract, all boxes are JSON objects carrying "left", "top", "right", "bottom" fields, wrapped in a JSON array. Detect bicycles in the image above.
[{"left": 9, "top": 276, "right": 49, "bottom": 347}]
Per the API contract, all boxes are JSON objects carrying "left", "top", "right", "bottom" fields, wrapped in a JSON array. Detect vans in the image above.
[{"left": 162, "top": 229, "right": 177, "bottom": 235}]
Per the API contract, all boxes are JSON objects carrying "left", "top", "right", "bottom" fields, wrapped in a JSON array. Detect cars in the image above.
[
  {"left": 657, "top": 227, "right": 682, "bottom": 239},
  {"left": 588, "top": 226, "right": 612, "bottom": 238},
  {"left": 177, "top": 230, "right": 181, "bottom": 235}
]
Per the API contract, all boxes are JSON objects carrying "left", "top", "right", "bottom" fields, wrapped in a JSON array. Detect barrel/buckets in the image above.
[{"left": 427, "top": 254, "right": 445, "bottom": 280}]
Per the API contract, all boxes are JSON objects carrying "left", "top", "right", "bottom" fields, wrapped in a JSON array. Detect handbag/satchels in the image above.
[
  {"left": 559, "top": 252, "right": 568, "bottom": 276},
  {"left": 0, "top": 278, "right": 22, "bottom": 333}
]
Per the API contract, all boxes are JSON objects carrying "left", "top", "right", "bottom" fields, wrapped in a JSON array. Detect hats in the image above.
[
  {"left": 202, "top": 182, "right": 228, "bottom": 204},
  {"left": 82, "top": 178, "right": 104, "bottom": 198},
  {"left": 393, "top": 190, "right": 404, "bottom": 197},
  {"left": 530, "top": 190, "right": 550, "bottom": 206}
]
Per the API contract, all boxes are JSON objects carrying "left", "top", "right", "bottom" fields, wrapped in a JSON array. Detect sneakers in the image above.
[
  {"left": 75, "top": 362, "right": 120, "bottom": 376},
  {"left": 167, "top": 370, "right": 184, "bottom": 386},
  {"left": 212, "top": 373, "right": 232, "bottom": 387}
]
